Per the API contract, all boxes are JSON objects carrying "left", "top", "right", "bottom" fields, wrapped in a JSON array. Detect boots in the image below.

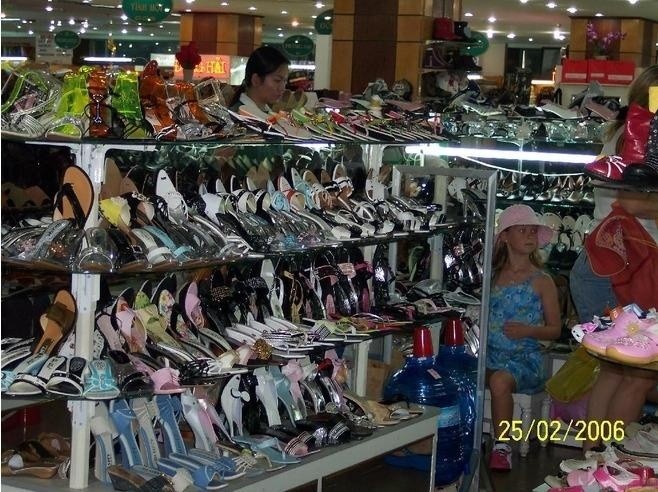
[
  {"left": 436, "top": 15, "right": 476, "bottom": 42},
  {"left": 582, "top": 103, "right": 657, "bottom": 189}
]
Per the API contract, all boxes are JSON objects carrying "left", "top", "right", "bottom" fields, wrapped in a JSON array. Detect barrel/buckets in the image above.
[
  {"left": 435, "top": 343, "right": 478, "bottom": 462},
  {"left": 382, "top": 354, "right": 465, "bottom": 486}
]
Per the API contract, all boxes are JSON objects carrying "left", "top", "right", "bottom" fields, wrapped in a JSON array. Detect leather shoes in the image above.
[{"left": 447, "top": 163, "right": 594, "bottom": 209}]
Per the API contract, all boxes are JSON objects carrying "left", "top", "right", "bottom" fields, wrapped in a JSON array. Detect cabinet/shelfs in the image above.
[{"left": 1, "top": 93, "right": 657, "bottom": 491}]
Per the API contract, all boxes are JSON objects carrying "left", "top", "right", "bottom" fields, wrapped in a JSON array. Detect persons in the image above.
[
  {"left": 567, "top": 62, "right": 657, "bottom": 458},
  {"left": 227, "top": 45, "right": 291, "bottom": 117},
  {"left": 480, "top": 204, "right": 563, "bottom": 473}
]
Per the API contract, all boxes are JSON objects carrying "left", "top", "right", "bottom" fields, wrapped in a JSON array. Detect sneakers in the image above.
[
  {"left": 489, "top": 441, "right": 514, "bottom": 470},
  {"left": 572, "top": 303, "right": 658, "bottom": 367}
]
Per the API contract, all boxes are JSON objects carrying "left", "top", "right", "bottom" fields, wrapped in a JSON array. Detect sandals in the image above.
[
  {"left": 91, "top": 160, "right": 489, "bottom": 405},
  {"left": 544, "top": 421, "right": 658, "bottom": 492},
  {"left": 2, "top": 165, "right": 115, "bottom": 273},
  {"left": 3, "top": 59, "right": 619, "bottom": 144},
  {"left": 545, "top": 211, "right": 594, "bottom": 268}
]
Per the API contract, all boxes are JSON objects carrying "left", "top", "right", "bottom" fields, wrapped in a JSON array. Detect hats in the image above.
[{"left": 495, "top": 203, "right": 553, "bottom": 250}]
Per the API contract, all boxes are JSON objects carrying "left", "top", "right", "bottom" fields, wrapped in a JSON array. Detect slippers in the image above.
[
  {"left": 87, "top": 360, "right": 118, "bottom": 401},
  {"left": 2, "top": 433, "right": 74, "bottom": 479},
  {"left": 37, "top": 289, "right": 78, "bottom": 357},
  {"left": 2, "top": 337, "right": 86, "bottom": 403}
]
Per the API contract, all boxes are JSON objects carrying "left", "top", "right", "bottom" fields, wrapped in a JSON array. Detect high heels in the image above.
[{"left": 89, "top": 401, "right": 424, "bottom": 490}]
